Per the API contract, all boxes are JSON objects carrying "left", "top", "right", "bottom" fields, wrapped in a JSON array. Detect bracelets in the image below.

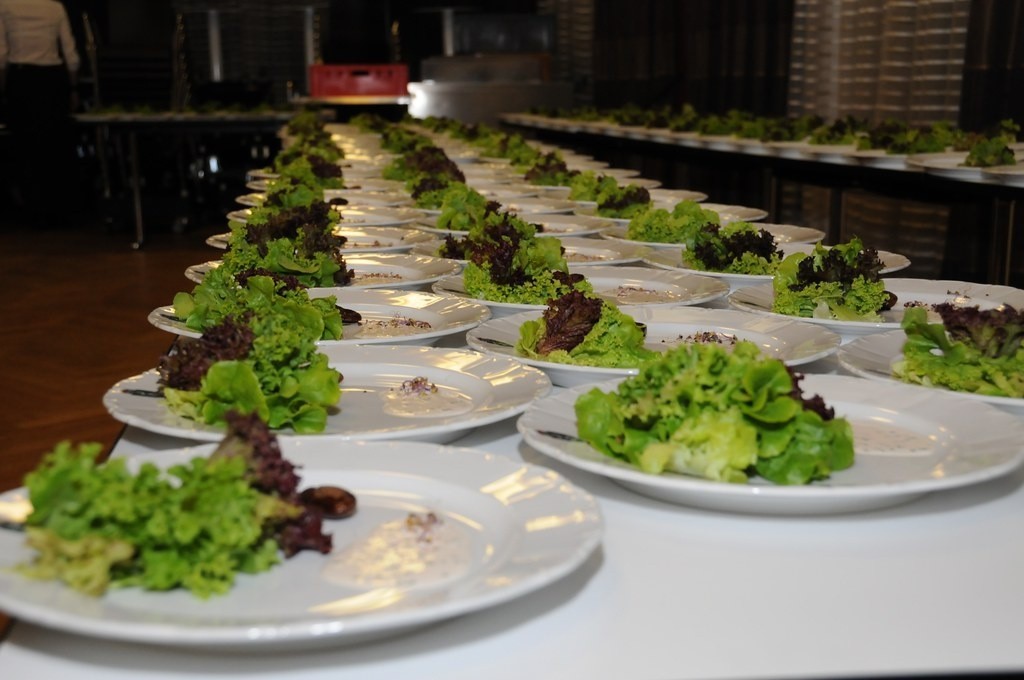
[{"left": 69, "top": 85, "right": 80, "bottom": 92}]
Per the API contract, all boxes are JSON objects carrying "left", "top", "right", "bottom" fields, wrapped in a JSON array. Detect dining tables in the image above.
[{"left": 0, "top": 109, "right": 1024, "bottom": 680}]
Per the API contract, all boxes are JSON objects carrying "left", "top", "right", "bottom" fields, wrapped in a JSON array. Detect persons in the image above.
[{"left": 0, "top": 0, "right": 82, "bottom": 229}]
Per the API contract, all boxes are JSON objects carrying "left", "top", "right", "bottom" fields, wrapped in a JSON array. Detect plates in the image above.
[{"left": 0, "top": 112, "right": 1024, "bottom": 653}]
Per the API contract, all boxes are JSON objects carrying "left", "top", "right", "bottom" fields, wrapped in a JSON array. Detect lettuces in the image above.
[{"left": 21, "top": 104, "right": 1024, "bottom": 600}]
